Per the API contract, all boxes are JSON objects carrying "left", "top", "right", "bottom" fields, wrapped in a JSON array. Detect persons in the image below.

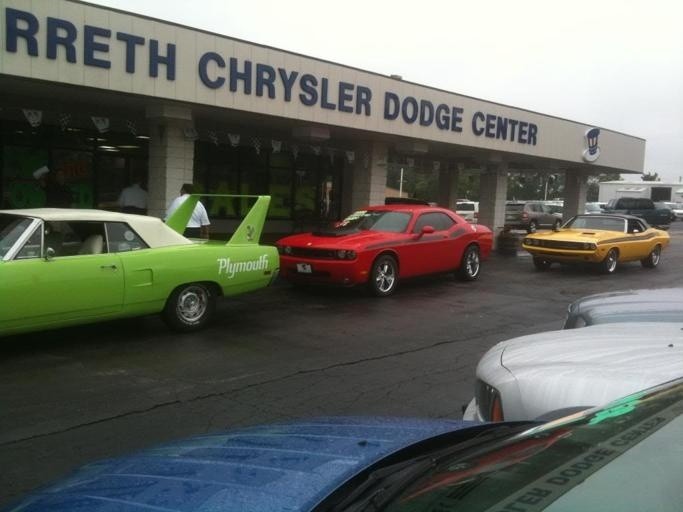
[
  {"left": 163, "top": 183, "right": 212, "bottom": 239},
  {"left": 40, "top": 168, "right": 74, "bottom": 208},
  {"left": 120, "top": 179, "right": 149, "bottom": 214}
]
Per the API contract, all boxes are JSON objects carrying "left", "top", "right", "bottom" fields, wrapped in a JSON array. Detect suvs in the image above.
[{"left": 455, "top": 199, "right": 683, "bottom": 233}]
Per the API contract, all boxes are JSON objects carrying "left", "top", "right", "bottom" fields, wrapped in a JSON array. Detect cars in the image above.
[
  {"left": 0, "top": 194, "right": 281, "bottom": 339},
  {"left": 521, "top": 213, "right": 672, "bottom": 274},
  {"left": 276, "top": 202, "right": 494, "bottom": 298}
]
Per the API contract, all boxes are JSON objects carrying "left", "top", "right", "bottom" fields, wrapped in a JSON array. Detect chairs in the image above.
[{"left": 76, "top": 235, "right": 103, "bottom": 254}]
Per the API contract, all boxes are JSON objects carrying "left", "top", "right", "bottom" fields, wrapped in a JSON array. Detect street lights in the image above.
[{"left": 542, "top": 174, "right": 556, "bottom": 200}]
